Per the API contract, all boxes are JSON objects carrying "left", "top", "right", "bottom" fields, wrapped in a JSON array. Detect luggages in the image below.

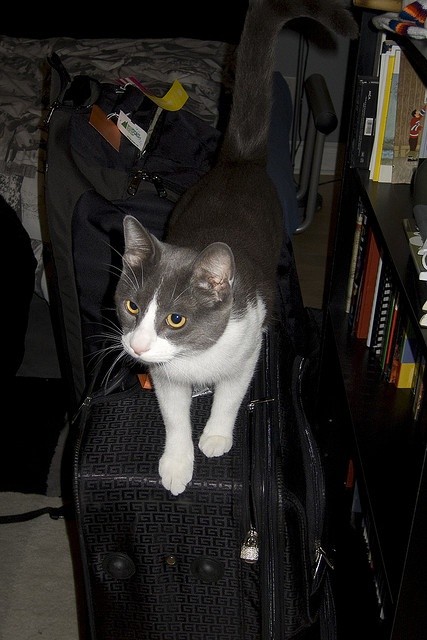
[{"left": 58, "top": 325, "right": 338, "bottom": 639}]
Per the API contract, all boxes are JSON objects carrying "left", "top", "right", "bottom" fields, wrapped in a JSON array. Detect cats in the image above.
[{"left": 84, "top": 0, "right": 363, "bottom": 498}]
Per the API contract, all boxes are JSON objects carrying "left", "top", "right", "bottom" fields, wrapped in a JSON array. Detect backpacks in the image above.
[{"left": 38, "top": 50, "right": 225, "bottom": 422}]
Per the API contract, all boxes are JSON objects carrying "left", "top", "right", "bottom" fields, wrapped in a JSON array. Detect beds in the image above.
[{"left": 0, "top": 36, "right": 302, "bottom": 380}]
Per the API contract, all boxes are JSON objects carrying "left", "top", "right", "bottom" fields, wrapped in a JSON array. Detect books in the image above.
[
  {"left": 349, "top": 32, "right": 427, "bottom": 185},
  {"left": 346, "top": 457, "right": 386, "bottom": 620},
  {"left": 344, "top": 195, "right": 426, "bottom": 420}
]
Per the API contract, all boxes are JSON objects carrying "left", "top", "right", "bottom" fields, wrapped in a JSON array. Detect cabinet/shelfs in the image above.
[{"left": 319, "top": 7, "right": 427, "bottom": 548}]
